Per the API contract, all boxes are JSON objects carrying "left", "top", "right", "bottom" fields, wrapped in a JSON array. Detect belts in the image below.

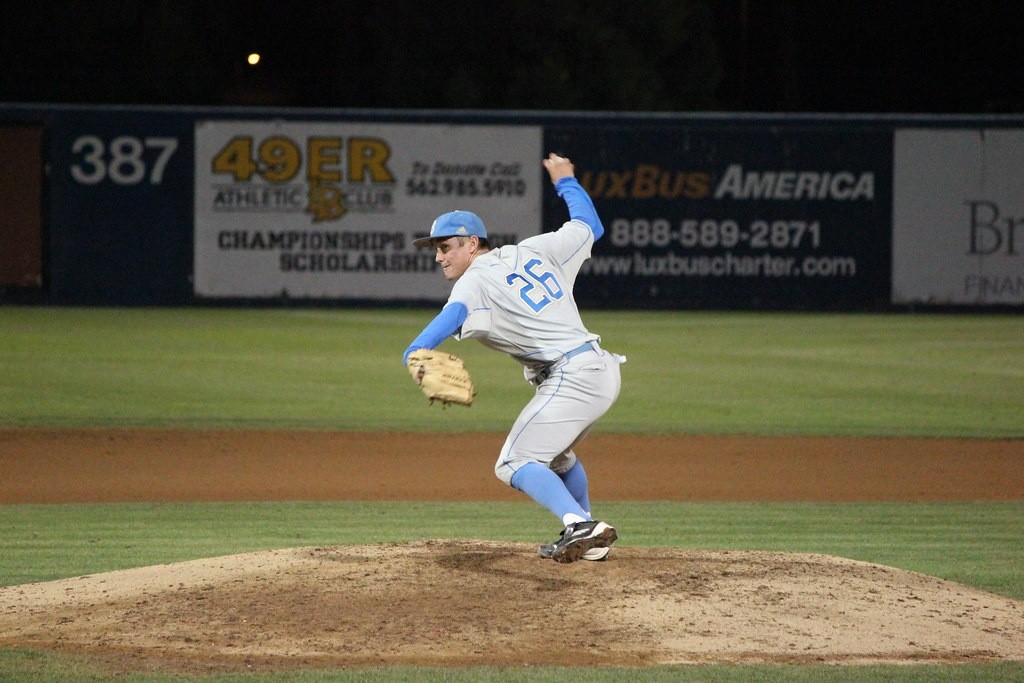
[{"left": 542, "top": 342, "right": 594, "bottom": 374}]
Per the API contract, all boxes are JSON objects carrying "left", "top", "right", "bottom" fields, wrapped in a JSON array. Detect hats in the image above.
[{"left": 411, "top": 209, "right": 488, "bottom": 249}]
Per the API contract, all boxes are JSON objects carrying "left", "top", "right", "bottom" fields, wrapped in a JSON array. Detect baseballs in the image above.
[{"left": 548, "top": 156, "right": 565, "bottom": 164}]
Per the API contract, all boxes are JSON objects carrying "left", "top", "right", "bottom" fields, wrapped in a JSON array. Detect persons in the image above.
[{"left": 403, "top": 153, "right": 621, "bottom": 561}]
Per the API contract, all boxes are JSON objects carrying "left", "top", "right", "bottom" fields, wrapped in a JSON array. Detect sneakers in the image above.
[{"left": 538, "top": 519, "right": 619, "bottom": 564}]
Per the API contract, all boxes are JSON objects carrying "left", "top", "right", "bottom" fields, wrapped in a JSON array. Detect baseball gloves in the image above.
[{"left": 407, "top": 344, "right": 477, "bottom": 410}]
